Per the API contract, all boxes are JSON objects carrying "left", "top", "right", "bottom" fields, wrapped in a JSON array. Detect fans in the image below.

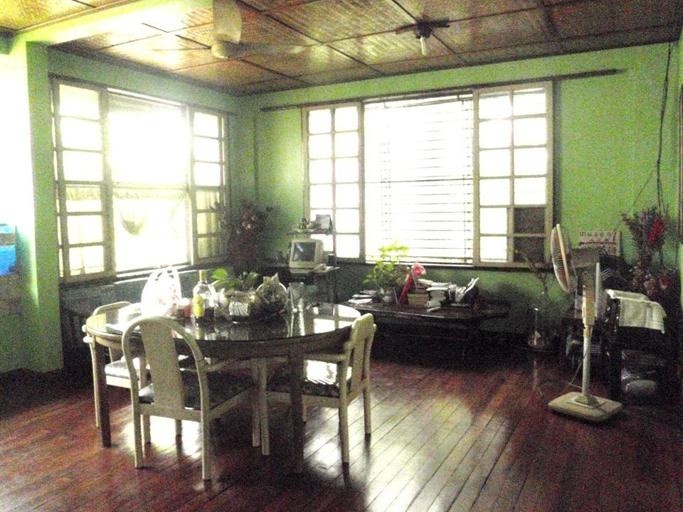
[
  {"left": 149, "top": 1, "right": 311, "bottom": 63},
  {"left": 544, "top": 224, "right": 626, "bottom": 427}
]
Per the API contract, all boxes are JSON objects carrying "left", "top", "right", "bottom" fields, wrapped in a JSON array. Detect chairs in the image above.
[
  {"left": 84, "top": 298, "right": 184, "bottom": 445},
  {"left": 121, "top": 315, "right": 261, "bottom": 482},
  {"left": 257, "top": 311, "right": 376, "bottom": 467}
]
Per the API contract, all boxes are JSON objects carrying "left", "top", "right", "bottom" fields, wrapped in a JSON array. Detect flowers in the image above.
[{"left": 621, "top": 207, "right": 674, "bottom": 301}]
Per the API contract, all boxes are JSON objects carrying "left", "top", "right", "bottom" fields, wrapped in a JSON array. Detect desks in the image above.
[{"left": 337, "top": 287, "right": 511, "bottom": 367}]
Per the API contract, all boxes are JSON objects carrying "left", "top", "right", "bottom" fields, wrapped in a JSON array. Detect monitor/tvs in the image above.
[{"left": 289, "top": 238, "right": 322, "bottom": 273}]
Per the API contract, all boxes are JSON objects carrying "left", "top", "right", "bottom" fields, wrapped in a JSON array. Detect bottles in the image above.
[
  {"left": 256, "top": 278, "right": 288, "bottom": 315},
  {"left": 528, "top": 286, "right": 558, "bottom": 350},
  {"left": 191, "top": 269, "right": 213, "bottom": 327}
]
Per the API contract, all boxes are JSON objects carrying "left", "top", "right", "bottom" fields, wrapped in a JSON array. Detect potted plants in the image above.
[
  {"left": 512, "top": 245, "right": 560, "bottom": 356},
  {"left": 364, "top": 244, "right": 410, "bottom": 303}
]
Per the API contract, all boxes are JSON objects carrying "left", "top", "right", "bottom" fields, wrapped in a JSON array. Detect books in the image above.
[{"left": 407, "top": 277, "right": 457, "bottom": 312}]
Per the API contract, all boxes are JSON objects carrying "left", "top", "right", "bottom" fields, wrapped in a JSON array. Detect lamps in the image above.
[{"left": 394, "top": 18, "right": 450, "bottom": 56}]
